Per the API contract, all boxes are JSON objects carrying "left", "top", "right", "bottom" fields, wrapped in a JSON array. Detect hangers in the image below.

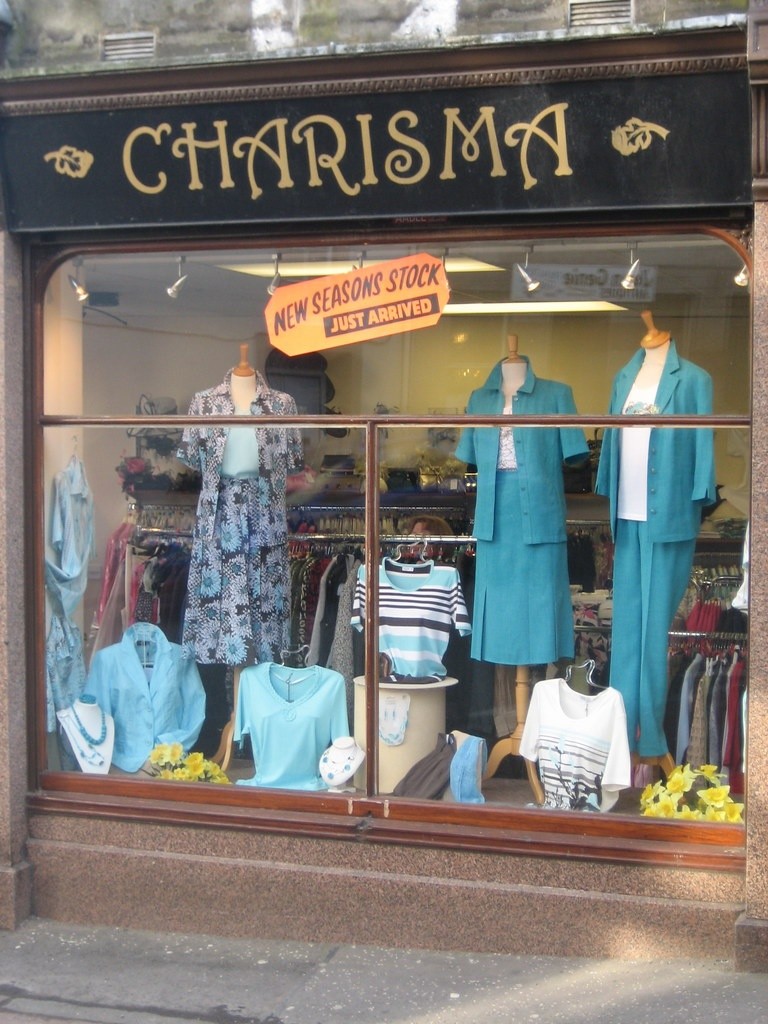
[
  {"left": 64, "top": 435, "right": 85, "bottom": 471},
  {"left": 478, "top": 518, "right": 750, "bottom": 662},
  {"left": 124, "top": 500, "right": 477, "bottom": 567}
]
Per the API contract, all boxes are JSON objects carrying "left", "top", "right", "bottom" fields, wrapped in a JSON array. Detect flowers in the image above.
[
  {"left": 640, "top": 763, "right": 744, "bottom": 824},
  {"left": 136, "top": 740, "right": 235, "bottom": 786}
]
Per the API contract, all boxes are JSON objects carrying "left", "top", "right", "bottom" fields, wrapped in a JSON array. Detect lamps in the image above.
[
  {"left": 514, "top": 245, "right": 539, "bottom": 294},
  {"left": 622, "top": 241, "right": 640, "bottom": 291},
  {"left": 68, "top": 259, "right": 89, "bottom": 303},
  {"left": 266, "top": 253, "right": 282, "bottom": 296},
  {"left": 733, "top": 265, "right": 749, "bottom": 288},
  {"left": 166, "top": 256, "right": 190, "bottom": 297}
]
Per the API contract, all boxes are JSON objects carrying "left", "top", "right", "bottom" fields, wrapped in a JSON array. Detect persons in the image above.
[
  {"left": 407, "top": 516, "right": 454, "bottom": 560},
  {"left": 178, "top": 367, "right": 308, "bottom": 667},
  {"left": 453, "top": 356, "right": 589, "bottom": 666},
  {"left": 593, "top": 330, "right": 716, "bottom": 758}
]
